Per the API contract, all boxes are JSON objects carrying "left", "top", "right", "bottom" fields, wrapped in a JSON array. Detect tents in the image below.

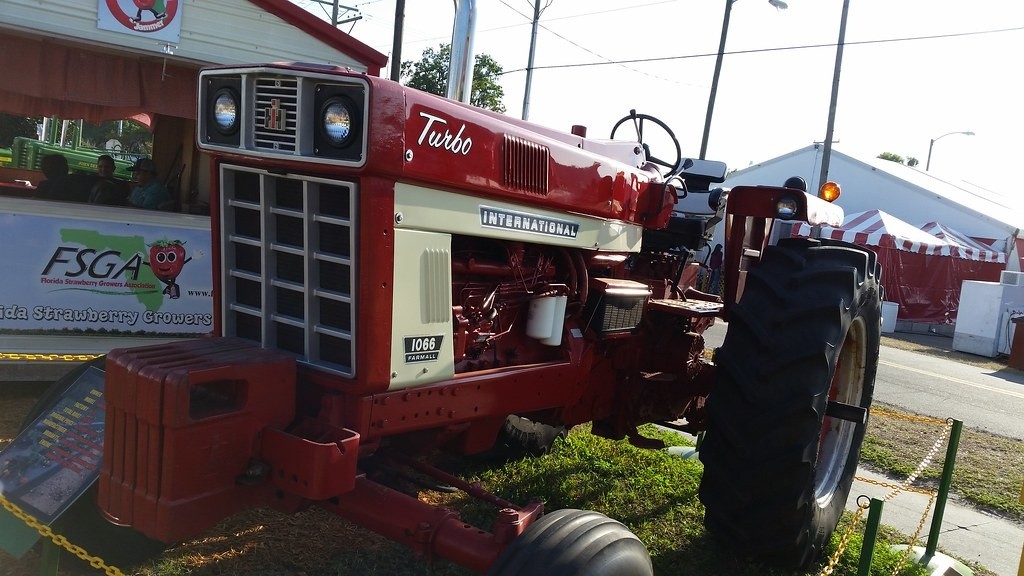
[
  {"left": 919, "top": 221, "right": 1006, "bottom": 324},
  {"left": 792, "top": 209, "right": 953, "bottom": 331}
]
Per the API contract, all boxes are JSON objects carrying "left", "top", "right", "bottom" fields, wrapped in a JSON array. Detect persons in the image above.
[{"left": 33, "top": 155, "right": 173, "bottom": 211}]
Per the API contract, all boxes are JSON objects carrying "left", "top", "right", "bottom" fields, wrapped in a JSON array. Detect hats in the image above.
[{"left": 125, "top": 158, "right": 156, "bottom": 174}]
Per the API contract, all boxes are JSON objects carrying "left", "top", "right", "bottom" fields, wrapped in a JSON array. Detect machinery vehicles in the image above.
[{"left": 92, "top": 56, "right": 888, "bottom": 576}]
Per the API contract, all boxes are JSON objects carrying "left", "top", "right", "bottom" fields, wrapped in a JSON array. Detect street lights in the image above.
[
  {"left": 925, "top": 131, "right": 976, "bottom": 172},
  {"left": 699, "top": 0, "right": 789, "bottom": 159}
]
